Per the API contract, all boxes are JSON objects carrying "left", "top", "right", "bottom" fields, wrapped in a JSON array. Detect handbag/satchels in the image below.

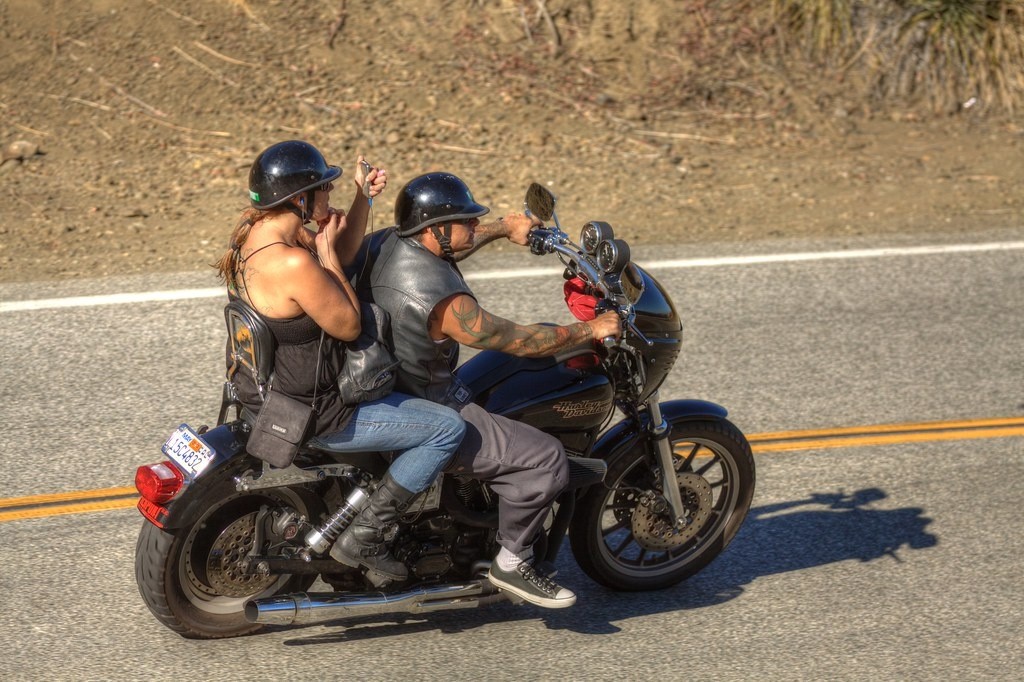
[{"left": 245, "top": 391, "right": 314, "bottom": 469}]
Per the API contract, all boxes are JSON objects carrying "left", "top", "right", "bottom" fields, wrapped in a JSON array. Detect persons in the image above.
[
  {"left": 351, "top": 172, "right": 621, "bottom": 608},
  {"left": 205, "top": 140, "right": 466, "bottom": 582}
]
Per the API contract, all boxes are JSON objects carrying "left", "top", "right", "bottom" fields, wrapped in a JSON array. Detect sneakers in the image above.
[{"left": 488, "top": 557, "right": 576, "bottom": 608}]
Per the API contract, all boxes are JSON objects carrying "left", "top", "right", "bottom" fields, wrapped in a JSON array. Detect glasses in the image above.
[
  {"left": 314, "top": 182, "right": 329, "bottom": 192},
  {"left": 458, "top": 218, "right": 470, "bottom": 224}
]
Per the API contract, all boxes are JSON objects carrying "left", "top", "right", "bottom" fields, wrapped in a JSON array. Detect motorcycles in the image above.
[{"left": 131, "top": 183, "right": 757, "bottom": 641}]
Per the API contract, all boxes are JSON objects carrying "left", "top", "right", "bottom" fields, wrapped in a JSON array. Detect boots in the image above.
[{"left": 329, "top": 469, "right": 421, "bottom": 581}]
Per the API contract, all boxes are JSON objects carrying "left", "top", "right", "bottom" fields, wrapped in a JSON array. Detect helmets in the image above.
[
  {"left": 394, "top": 172, "right": 490, "bottom": 237},
  {"left": 248, "top": 140, "right": 342, "bottom": 211}
]
